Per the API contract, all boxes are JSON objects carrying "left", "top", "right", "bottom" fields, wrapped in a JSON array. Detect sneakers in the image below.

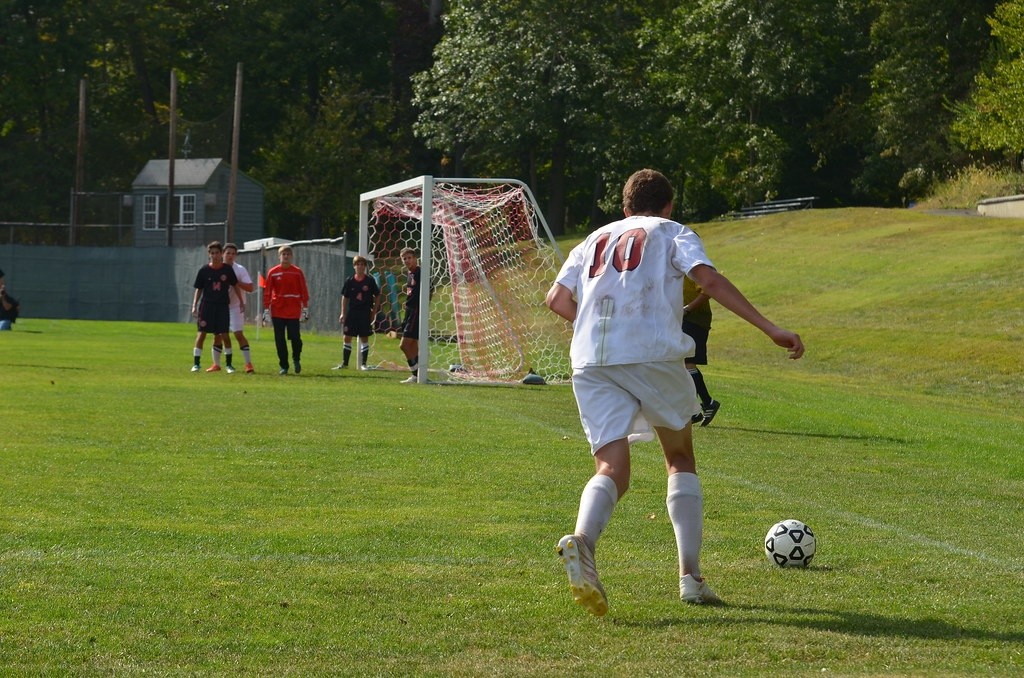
[
  {"left": 279, "top": 368, "right": 287, "bottom": 374},
  {"left": 245, "top": 363, "right": 253, "bottom": 373},
  {"left": 331, "top": 365, "right": 349, "bottom": 371},
  {"left": 206, "top": 364, "right": 221, "bottom": 372},
  {"left": 400, "top": 375, "right": 417, "bottom": 383},
  {"left": 679, "top": 573, "right": 722, "bottom": 604},
  {"left": 361, "top": 365, "right": 370, "bottom": 371},
  {"left": 294, "top": 360, "right": 301, "bottom": 373},
  {"left": 700, "top": 400, "right": 720, "bottom": 427},
  {"left": 692, "top": 411, "right": 703, "bottom": 424},
  {"left": 192, "top": 365, "right": 199, "bottom": 372},
  {"left": 556, "top": 535, "right": 608, "bottom": 616},
  {"left": 226, "top": 365, "right": 234, "bottom": 373}
]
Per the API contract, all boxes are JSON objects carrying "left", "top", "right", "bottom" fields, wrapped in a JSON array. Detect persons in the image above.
[
  {"left": 190, "top": 242, "right": 245, "bottom": 373},
  {"left": 546, "top": 170, "right": 805, "bottom": 615},
  {"left": 682, "top": 231, "right": 720, "bottom": 426},
  {"left": 332, "top": 256, "right": 380, "bottom": 371},
  {"left": 264, "top": 246, "right": 309, "bottom": 374},
  {"left": 399, "top": 248, "right": 434, "bottom": 383},
  {"left": 206, "top": 244, "right": 254, "bottom": 373},
  {"left": 0, "top": 270, "right": 20, "bottom": 330}
]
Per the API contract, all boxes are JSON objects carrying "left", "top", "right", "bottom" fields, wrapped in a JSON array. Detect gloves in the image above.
[
  {"left": 263, "top": 309, "right": 271, "bottom": 321},
  {"left": 299, "top": 308, "right": 309, "bottom": 322}
]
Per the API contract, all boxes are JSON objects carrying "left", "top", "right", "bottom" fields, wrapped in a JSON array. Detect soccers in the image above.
[{"left": 764, "top": 519, "right": 816, "bottom": 568}]
